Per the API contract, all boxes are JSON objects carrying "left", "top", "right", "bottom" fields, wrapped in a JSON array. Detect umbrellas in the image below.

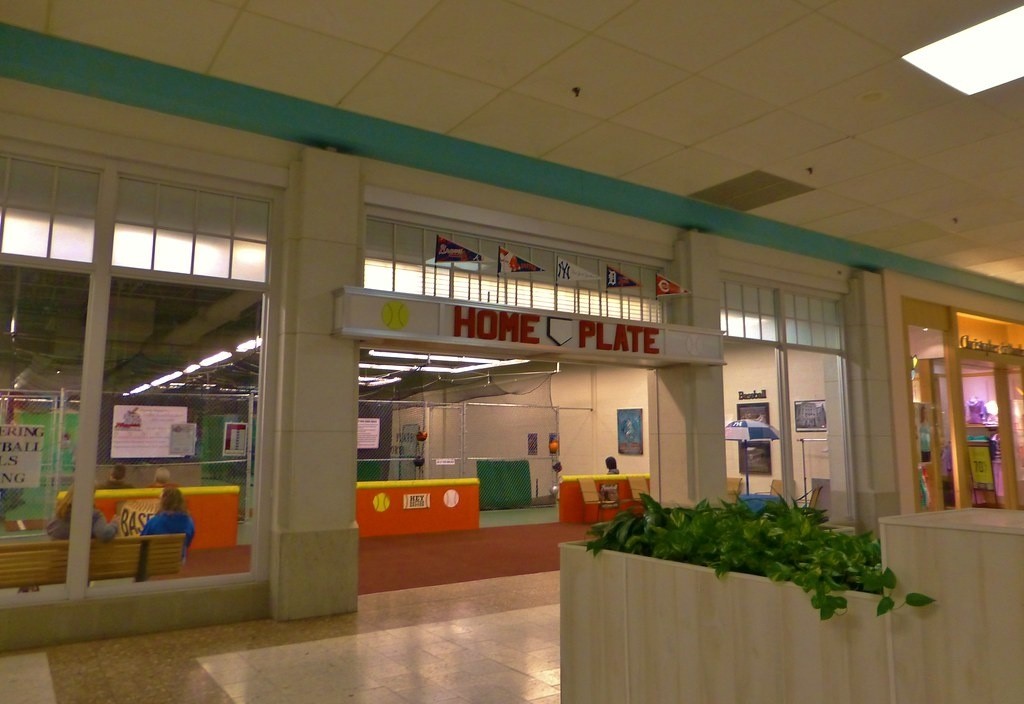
[{"left": 726, "top": 419, "right": 780, "bottom": 495}]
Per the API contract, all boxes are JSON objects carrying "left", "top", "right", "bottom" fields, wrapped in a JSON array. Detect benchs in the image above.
[
  {"left": 559, "top": 474, "right": 650, "bottom": 525},
  {"left": 0, "top": 534, "right": 187, "bottom": 590},
  {"left": 356, "top": 479, "right": 481, "bottom": 537},
  {"left": 56, "top": 485, "right": 240, "bottom": 549}
]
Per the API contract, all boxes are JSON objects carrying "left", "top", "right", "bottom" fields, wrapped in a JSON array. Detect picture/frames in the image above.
[
  {"left": 736, "top": 402, "right": 772, "bottom": 475},
  {"left": 794, "top": 400, "right": 827, "bottom": 432}
]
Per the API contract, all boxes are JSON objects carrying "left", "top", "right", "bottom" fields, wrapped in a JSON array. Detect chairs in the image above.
[
  {"left": 727, "top": 478, "right": 744, "bottom": 505},
  {"left": 755, "top": 479, "right": 781, "bottom": 495},
  {"left": 808, "top": 486, "right": 823, "bottom": 508}
]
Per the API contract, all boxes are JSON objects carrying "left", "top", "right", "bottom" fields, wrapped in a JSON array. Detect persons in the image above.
[
  {"left": 96, "top": 464, "right": 135, "bottom": 489},
  {"left": 145, "top": 467, "right": 173, "bottom": 488},
  {"left": 2, "top": 482, "right": 121, "bottom": 593},
  {"left": 139, "top": 486, "right": 195, "bottom": 564},
  {"left": 967, "top": 397, "right": 983, "bottom": 424},
  {"left": 919, "top": 408, "right": 932, "bottom": 461},
  {"left": 605, "top": 456, "right": 619, "bottom": 474}
]
